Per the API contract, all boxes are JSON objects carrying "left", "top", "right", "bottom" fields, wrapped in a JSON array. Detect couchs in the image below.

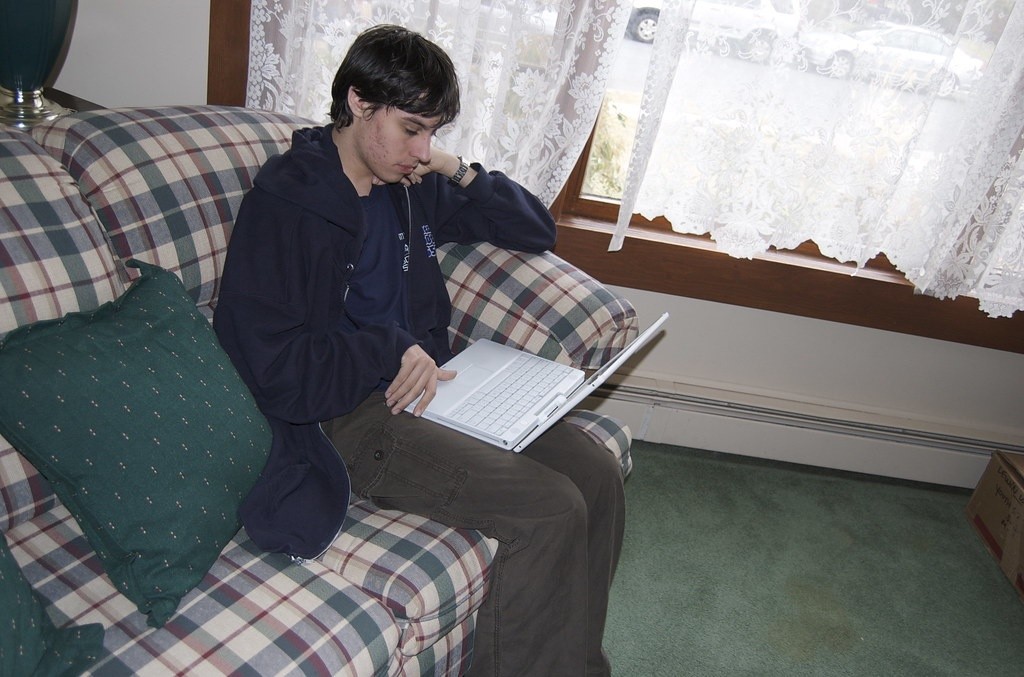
[{"left": 0, "top": 103, "right": 640, "bottom": 677}]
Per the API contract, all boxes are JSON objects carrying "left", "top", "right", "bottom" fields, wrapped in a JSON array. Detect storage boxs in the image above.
[{"left": 967, "top": 449, "right": 1024, "bottom": 601}]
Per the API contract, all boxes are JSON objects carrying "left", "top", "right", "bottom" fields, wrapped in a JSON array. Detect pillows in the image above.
[
  {"left": 0, "top": 534, "right": 106, "bottom": 677},
  {"left": 0, "top": 256, "right": 277, "bottom": 631}
]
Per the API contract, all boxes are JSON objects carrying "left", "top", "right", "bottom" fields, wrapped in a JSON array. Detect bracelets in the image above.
[{"left": 450, "top": 155, "right": 471, "bottom": 184}]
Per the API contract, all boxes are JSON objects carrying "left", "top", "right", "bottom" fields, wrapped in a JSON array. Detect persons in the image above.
[{"left": 211, "top": 23, "right": 628, "bottom": 677}]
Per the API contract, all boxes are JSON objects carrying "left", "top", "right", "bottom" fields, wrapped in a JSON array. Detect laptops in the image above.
[{"left": 403, "top": 312, "right": 670, "bottom": 452}]
